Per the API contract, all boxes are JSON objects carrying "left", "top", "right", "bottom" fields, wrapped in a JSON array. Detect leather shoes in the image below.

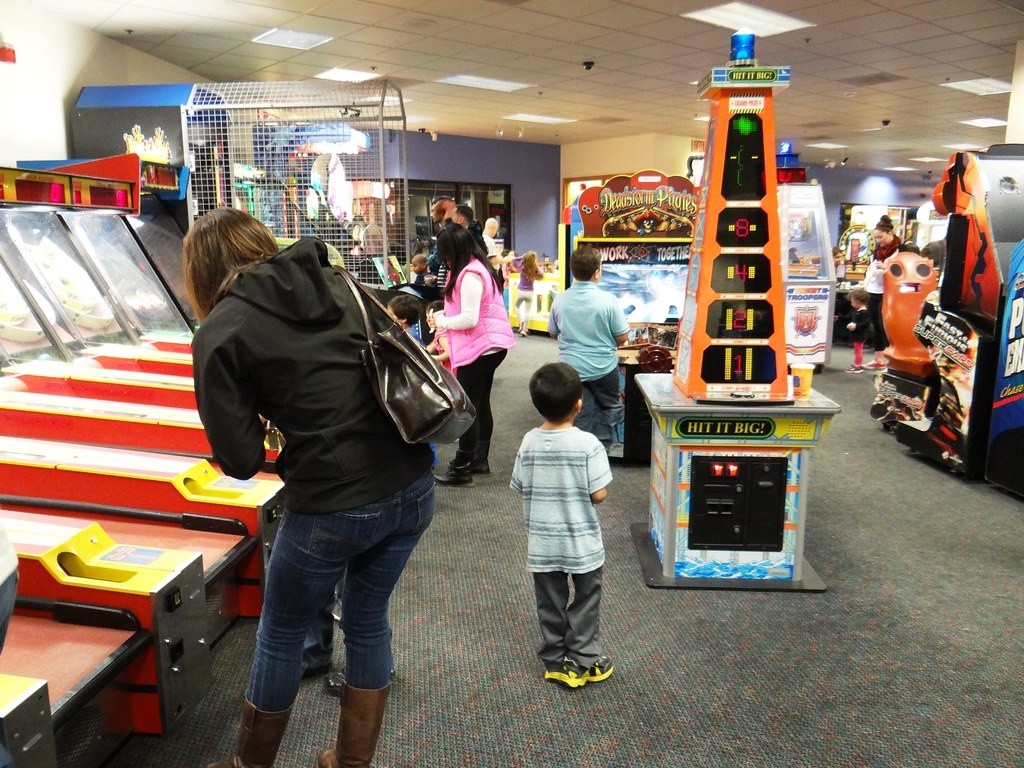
[{"left": 325, "top": 668, "right": 395, "bottom": 693}]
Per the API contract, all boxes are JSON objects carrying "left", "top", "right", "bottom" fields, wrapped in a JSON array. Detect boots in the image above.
[
  {"left": 318, "top": 679, "right": 390, "bottom": 768},
  {"left": 472, "top": 440, "right": 491, "bottom": 478},
  {"left": 433, "top": 450, "right": 475, "bottom": 488},
  {"left": 207, "top": 688, "right": 294, "bottom": 768}
]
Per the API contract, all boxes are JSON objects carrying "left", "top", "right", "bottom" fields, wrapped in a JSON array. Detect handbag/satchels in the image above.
[{"left": 332, "top": 265, "right": 477, "bottom": 444}]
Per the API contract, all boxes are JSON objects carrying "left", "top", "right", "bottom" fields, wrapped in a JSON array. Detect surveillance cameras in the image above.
[
  {"left": 418, "top": 129, "right": 425, "bottom": 133},
  {"left": 882, "top": 120, "right": 890, "bottom": 126},
  {"left": 583, "top": 62, "right": 594, "bottom": 70}
]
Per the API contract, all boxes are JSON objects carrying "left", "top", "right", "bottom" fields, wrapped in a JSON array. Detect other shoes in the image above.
[{"left": 521, "top": 329, "right": 528, "bottom": 336}]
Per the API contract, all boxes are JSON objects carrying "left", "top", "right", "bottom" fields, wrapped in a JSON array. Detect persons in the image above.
[
  {"left": 482, "top": 217, "right": 515, "bottom": 277},
  {"left": 844, "top": 287, "right": 870, "bottom": 375},
  {"left": 787, "top": 248, "right": 800, "bottom": 264},
  {"left": 426, "top": 221, "right": 517, "bottom": 488},
  {"left": 861, "top": 213, "right": 907, "bottom": 371},
  {"left": 452, "top": 204, "right": 489, "bottom": 259},
  {"left": 181, "top": 207, "right": 440, "bottom": 768},
  {"left": 546, "top": 246, "right": 630, "bottom": 459},
  {"left": 385, "top": 294, "right": 439, "bottom": 472},
  {"left": 423, "top": 299, "right": 457, "bottom": 380},
  {"left": 512, "top": 251, "right": 544, "bottom": 337},
  {"left": 831, "top": 247, "right": 842, "bottom": 275},
  {"left": 508, "top": 363, "right": 615, "bottom": 690},
  {"left": 425, "top": 197, "right": 457, "bottom": 278},
  {"left": 411, "top": 254, "right": 430, "bottom": 286}
]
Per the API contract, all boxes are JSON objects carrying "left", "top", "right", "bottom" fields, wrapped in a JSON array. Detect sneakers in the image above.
[
  {"left": 559, "top": 656, "right": 614, "bottom": 688},
  {"left": 844, "top": 365, "right": 861, "bottom": 374},
  {"left": 544, "top": 668, "right": 561, "bottom": 682},
  {"left": 859, "top": 366, "right": 863, "bottom": 372},
  {"left": 863, "top": 362, "right": 887, "bottom": 369}
]
